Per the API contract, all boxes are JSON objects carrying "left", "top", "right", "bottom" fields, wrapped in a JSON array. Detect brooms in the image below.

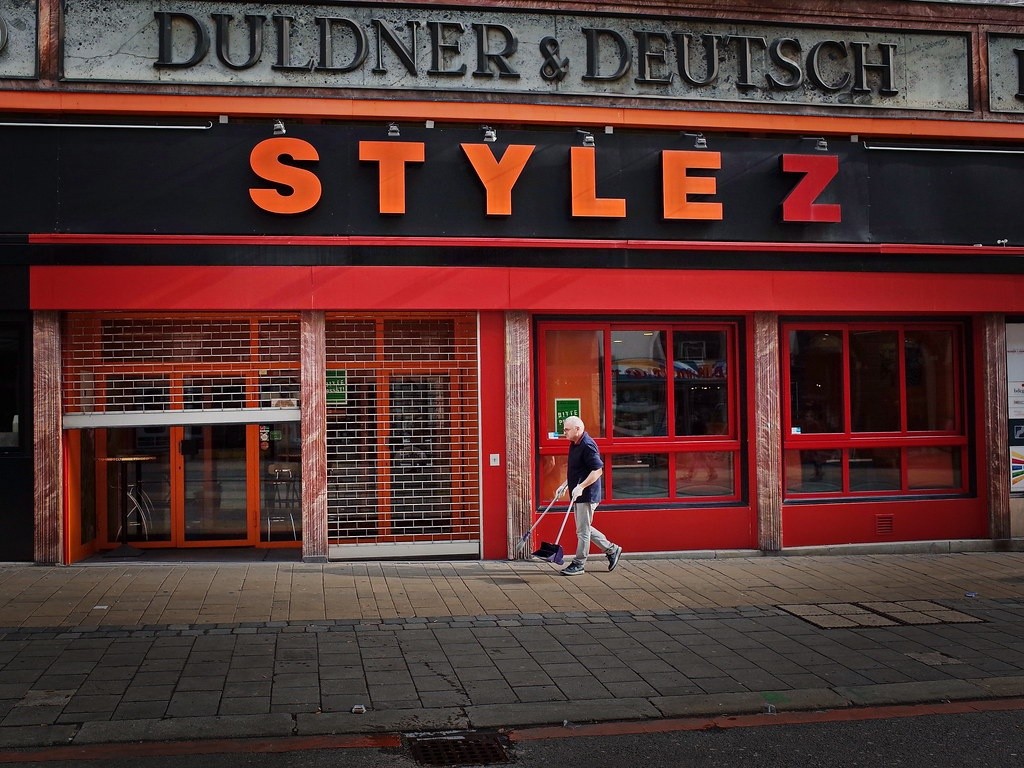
[{"left": 515, "top": 482, "right": 569, "bottom": 554}]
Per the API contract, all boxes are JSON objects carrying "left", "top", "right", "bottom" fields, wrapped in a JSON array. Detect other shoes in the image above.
[
  {"left": 707, "top": 474, "right": 719, "bottom": 483},
  {"left": 680, "top": 475, "right": 692, "bottom": 480}
]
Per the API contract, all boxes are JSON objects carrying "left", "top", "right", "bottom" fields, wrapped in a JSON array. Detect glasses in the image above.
[{"left": 563, "top": 428, "right": 578, "bottom": 432}]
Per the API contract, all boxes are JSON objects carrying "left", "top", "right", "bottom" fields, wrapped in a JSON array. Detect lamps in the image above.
[
  {"left": 273, "top": 120, "right": 286, "bottom": 134},
  {"left": 387, "top": 124, "right": 400, "bottom": 137},
  {"left": 482, "top": 126, "right": 497, "bottom": 142},
  {"left": 803, "top": 138, "right": 828, "bottom": 151},
  {"left": 576, "top": 130, "right": 596, "bottom": 147},
  {"left": 685, "top": 133, "right": 707, "bottom": 149}
]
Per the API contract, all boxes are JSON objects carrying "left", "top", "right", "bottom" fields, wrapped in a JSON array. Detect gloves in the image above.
[
  {"left": 572, "top": 484, "right": 584, "bottom": 502},
  {"left": 556, "top": 486, "right": 567, "bottom": 502}
]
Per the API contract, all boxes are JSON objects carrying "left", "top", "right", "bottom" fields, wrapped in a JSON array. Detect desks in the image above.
[
  {"left": 261, "top": 480, "right": 300, "bottom": 543},
  {"left": 93, "top": 456, "right": 156, "bottom": 557}
]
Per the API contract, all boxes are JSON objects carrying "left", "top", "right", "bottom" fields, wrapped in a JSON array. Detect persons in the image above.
[
  {"left": 680, "top": 412, "right": 718, "bottom": 482},
  {"left": 136, "top": 440, "right": 201, "bottom": 532},
  {"left": 556, "top": 416, "right": 623, "bottom": 575}
]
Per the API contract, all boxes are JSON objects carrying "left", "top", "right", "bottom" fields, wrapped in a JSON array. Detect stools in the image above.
[
  {"left": 108, "top": 464, "right": 155, "bottom": 543},
  {"left": 268, "top": 463, "right": 300, "bottom": 526}
]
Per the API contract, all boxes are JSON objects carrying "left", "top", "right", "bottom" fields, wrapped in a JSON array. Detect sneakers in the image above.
[
  {"left": 606, "top": 546, "right": 623, "bottom": 571},
  {"left": 561, "top": 562, "right": 585, "bottom": 575}
]
